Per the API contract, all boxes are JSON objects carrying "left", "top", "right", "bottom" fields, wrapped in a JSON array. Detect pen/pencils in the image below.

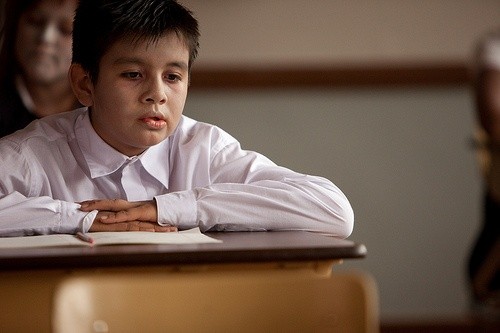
[{"left": 75, "top": 230, "right": 95, "bottom": 245}]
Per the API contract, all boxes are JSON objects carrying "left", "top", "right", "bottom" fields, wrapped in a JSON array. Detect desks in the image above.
[{"left": 0, "top": 230, "right": 368, "bottom": 333}]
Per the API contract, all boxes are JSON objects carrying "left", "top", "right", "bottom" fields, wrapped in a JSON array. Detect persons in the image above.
[
  {"left": 0, "top": 0, "right": 354, "bottom": 237},
  {"left": 0, "top": 0, "right": 85, "bottom": 137}
]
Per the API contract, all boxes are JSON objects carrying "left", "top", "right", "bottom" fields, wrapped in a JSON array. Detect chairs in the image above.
[{"left": 49, "top": 270, "right": 380, "bottom": 333}]
